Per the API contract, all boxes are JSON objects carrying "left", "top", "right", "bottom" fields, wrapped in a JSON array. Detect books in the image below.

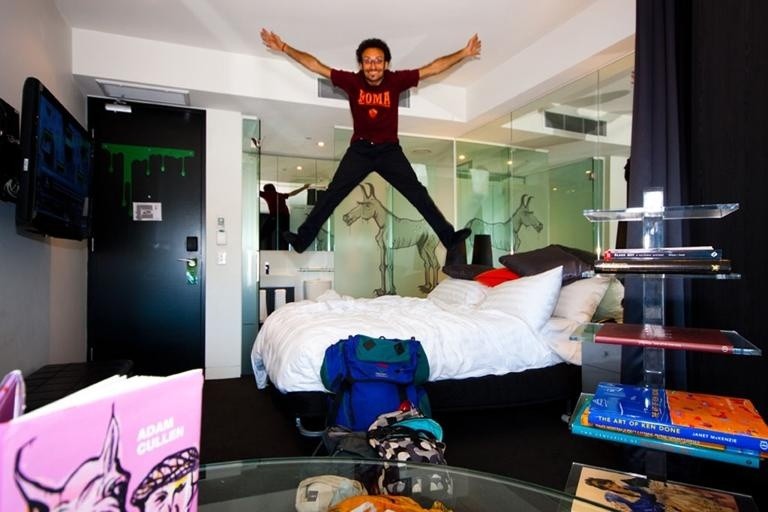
[{"left": 0, "top": 367, "right": 207, "bottom": 512}]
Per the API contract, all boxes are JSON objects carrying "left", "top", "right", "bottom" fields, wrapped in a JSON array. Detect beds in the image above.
[{"left": 251, "top": 243, "right": 627, "bottom": 443}]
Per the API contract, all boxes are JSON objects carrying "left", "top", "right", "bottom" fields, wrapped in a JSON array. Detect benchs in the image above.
[{"left": 9, "top": 359, "right": 135, "bottom": 415}]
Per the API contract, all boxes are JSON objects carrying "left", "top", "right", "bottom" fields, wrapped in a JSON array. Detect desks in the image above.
[{"left": 195, "top": 462, "right": 621, "bottom": 512}]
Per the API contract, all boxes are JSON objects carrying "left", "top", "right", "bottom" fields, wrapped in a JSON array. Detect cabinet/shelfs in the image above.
[{"left": 556, "top": 190, "right": 767, "bottom": 512}]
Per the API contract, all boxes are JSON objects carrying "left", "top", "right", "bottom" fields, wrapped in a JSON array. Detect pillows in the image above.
[{"left": 427, "top": 244, "right": 625, "bottom": 335}]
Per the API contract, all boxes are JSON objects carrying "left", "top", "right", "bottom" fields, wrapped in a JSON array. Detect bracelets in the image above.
[{"left": 281, "top": 43, "right": 286, "bottom": 52}]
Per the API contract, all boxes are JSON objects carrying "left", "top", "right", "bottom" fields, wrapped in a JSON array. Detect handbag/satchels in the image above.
[{"left": 295, "top": 335, "right": 452, "bottom": 512}]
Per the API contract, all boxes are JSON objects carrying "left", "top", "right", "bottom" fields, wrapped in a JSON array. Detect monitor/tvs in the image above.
[{"left": 15, "top": 77, "right": 95, "bottom": 241}]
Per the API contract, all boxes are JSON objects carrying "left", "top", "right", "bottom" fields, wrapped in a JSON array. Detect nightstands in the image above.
[{"left": 581, "top": 342, "right": 622, "bottom": 390}]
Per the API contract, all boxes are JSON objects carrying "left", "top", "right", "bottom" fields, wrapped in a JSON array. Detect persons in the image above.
[
  {"left": 260, "top": 28, "right": 482, "bottom": 254},
  {"left": 259, "top": 183, "right": 310, "bottom": 249},
  {"left": 584, "top": 476, "right": 735, "bottom": 512}
]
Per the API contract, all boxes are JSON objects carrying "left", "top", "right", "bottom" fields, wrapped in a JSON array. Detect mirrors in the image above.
[
  {"left": 455, "top": 140, "right": 550, "bottom": 268},
  {"left": 550, "top": 157, "right": 605, "bottom": 256},
  {"left": 259, "top": 153, "right": 335, "bottom": 252}
]
